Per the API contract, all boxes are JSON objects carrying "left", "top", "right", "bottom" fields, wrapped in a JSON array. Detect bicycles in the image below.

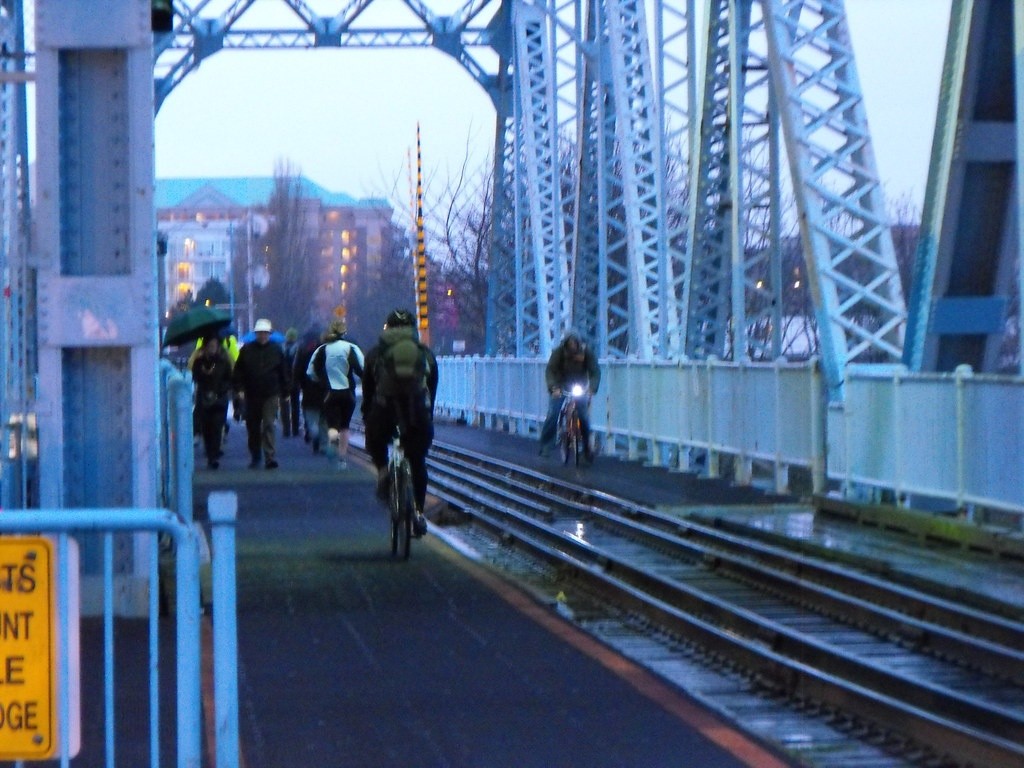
[
  {"left": 360, "top": 410, "right": 434, "bottom": 559},
  {"left": 544, "top": 383, "right": 595, "bottom": 470}
]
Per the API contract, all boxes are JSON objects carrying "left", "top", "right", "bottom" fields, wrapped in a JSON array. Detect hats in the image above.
[
  {"left": 387, "top": 309, "right": 415, "bottom": 327},
  {"left": 253, "top": 318, "right": 273, "bottom": 333}
]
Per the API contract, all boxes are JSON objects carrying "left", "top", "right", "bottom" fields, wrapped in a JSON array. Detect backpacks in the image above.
[{"left": 380, "top": 326, "right": 428, "bottom": 398}]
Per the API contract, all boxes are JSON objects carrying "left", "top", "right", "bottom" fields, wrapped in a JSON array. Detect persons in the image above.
[
  {"left": 361, "top": 308, "right": 438, "bottom": 536},
  {"left": 186, "top": 334, "right": 234, "bottom": 469},
  {"left": 233, "top": 319, "right": 289, "bottom": 469},
  {"left": 540, "top": 332, "right": 601, "bottom": 463},
  {"left": 290, "top": 323, "right": 322, "bottom": 452},
  {"left": 280, "top": 327, "right": 300, "bottom": 438},
  {"left": 196, "top": 335, "right": 239, "bottom": 433},
  {"left": 306, "top": 321, "right": 365, "bottom": 470}
]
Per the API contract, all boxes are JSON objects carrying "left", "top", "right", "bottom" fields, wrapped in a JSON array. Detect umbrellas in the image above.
[{"left": 161, "top": 306, "right": 234, "bottom": 347}]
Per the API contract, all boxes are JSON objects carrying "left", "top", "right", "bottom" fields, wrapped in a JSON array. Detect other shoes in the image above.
[
  {"left": 207, "top": 460, "right": 219, "bottom": 469},
  {"left": 413, "top": 512, "right": 427, "bottom": 535},
  {"left": 293, "top": 430, "right": 299, "bottom": 436},
  {"left": 327, "top": 438, "right": 337, "bottom": 456},
  {"left": 337, "top": 460, "right": 346, "bottom": 471},
  {"left": 265, "top": 461, "right": 277, "bottom": 469},
  {"left": 304, "top": 428, "right": 312, "bottom": 443},
  {"left": 377, "top": 473, "right": 389, "bottom": 503},
  {"left": 312, "top": 435, "right": 320, "bottom": 452},
  {"left": 249, "top": 459, "right": 262, "bottom": 468},
  {"left": 284, "top": 433, "right": 290, "bottom": 437}
]
